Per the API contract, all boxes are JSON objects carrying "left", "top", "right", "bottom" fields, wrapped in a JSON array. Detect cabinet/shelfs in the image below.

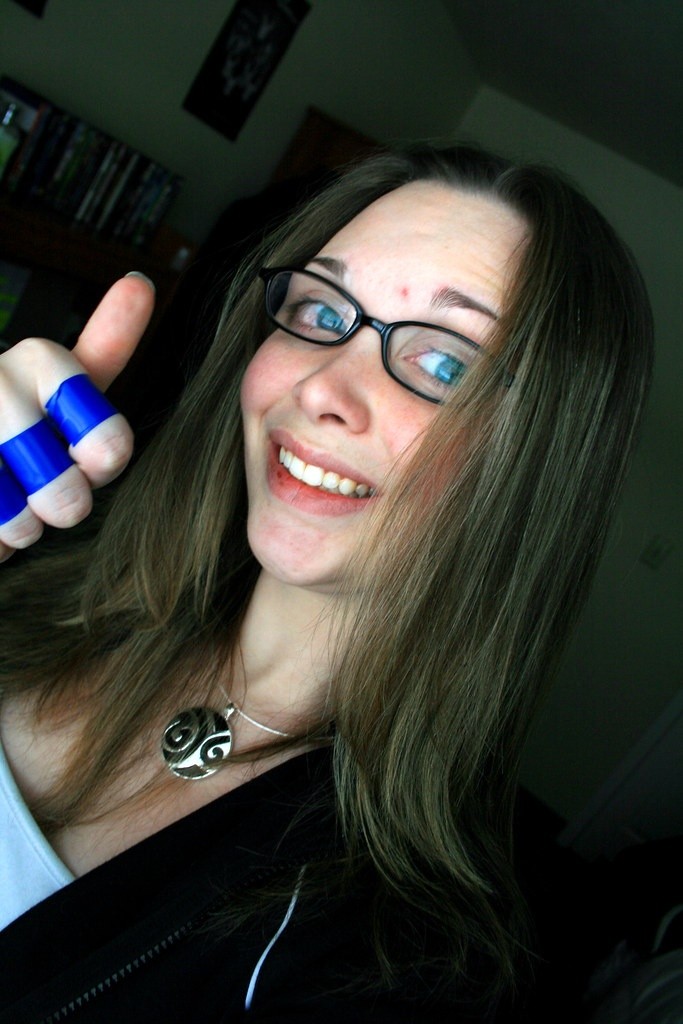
[{"left": 0, "top": 73, "right": 190, "bottom": 422}]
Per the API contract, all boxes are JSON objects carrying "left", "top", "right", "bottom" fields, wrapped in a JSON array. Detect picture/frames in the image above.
[{"left": 180, "top": 0, "right": 313, "bottom": 142}]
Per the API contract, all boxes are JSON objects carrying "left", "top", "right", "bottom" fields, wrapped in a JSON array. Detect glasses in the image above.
[{"left": 258, "top": 266, "right": 516, "bottom": 408}]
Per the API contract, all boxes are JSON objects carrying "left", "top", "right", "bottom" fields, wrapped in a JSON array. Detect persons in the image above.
[{"left": 0, "top": 141, "right": 650, "bottom": 1024}]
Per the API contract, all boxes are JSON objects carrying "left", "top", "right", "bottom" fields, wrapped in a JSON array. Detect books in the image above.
[{"left": 0, "top": 83, "right": 184, "bottom": 247}]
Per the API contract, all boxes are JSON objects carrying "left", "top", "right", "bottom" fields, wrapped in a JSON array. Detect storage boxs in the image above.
[{"left": 277, "top": 103, "right": 374, "bottom": 185}]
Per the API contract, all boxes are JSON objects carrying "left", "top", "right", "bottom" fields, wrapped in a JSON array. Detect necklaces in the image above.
[{"left": 161, "top": 648, "right": 334, "bottom": 783}]
